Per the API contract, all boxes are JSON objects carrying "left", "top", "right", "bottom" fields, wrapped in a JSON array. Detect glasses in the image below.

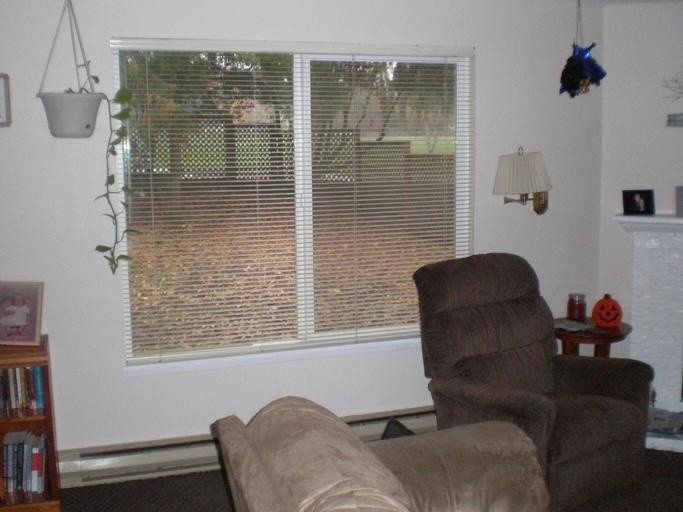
[{"left": 491, "top": 146, "right": 552, "bottom": 213}]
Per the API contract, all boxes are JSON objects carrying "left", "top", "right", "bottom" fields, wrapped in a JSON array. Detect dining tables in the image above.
[{"left": 35, "top": 60, "right": 142, "bottom": 274}]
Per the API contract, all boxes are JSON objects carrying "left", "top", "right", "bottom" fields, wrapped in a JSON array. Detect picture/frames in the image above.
[{"left": 552, "top": 316, "right": 632, "bottom": 358}]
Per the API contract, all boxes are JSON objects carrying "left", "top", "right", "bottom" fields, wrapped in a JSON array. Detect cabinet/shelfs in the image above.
[{"left": 567, "top": 293, "right": 586, "bottom": 322}]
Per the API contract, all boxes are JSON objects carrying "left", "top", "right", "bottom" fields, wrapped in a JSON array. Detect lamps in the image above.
[{"left": 411, "top": 251, "right": 654, "bottom": 512}]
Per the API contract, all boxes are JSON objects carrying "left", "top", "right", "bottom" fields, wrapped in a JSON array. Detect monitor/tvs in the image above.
[{"left": 0, "top": 366, "right": 48, "bottom": 506}]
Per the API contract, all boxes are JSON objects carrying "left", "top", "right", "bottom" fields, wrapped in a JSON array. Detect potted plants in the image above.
[
  {"left": 0, "top": 72, "right": 12, "bottom": 127},
  {"left": 0, "top": 281, "right": 43, "bottom": 346}
]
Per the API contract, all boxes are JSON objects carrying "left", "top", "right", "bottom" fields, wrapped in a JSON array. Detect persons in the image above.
[
  {"left": 627, "top": 194, "right": 650, "bottom": 215},
  {"left": 0, "top": 295, "right": 30, "bottom": 337}
]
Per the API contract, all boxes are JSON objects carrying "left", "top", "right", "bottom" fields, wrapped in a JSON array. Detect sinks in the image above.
[{"left": 210, "top": 394, "right": 552, "bottom": 512}]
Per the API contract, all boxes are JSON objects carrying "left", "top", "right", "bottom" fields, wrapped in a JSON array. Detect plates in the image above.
[{"left": 0, "top": 334, "right": 61, "bottom": 512}]
